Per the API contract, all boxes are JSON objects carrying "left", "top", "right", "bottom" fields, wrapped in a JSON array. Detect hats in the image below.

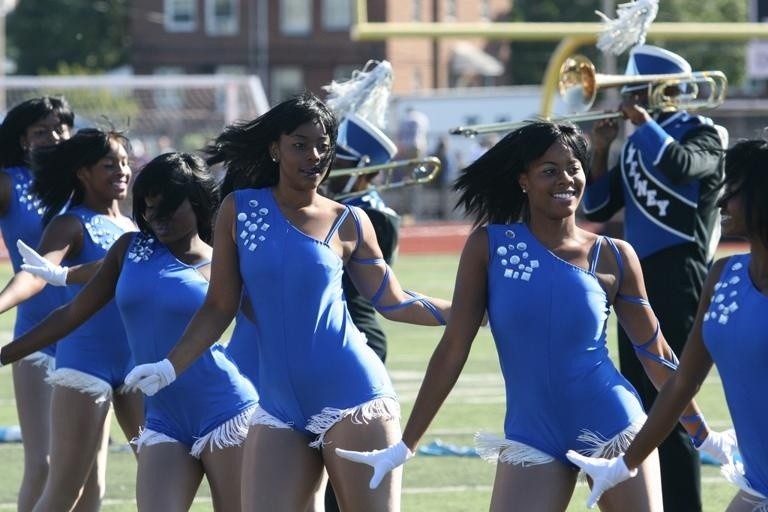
[
  {"left": 335, "top": 110, "right": 399, "bottom": 166},
  {"left": 621, "top": 43, "right": 694, "bottom": 98}
]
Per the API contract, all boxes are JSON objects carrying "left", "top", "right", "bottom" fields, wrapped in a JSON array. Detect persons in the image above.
[
  {"left": 0, "top": 129, "right": 167, "bottom": 512},
  {"left": 564, "top": 125, "right": 768, "bottom": 512},
  {"left": 335, "top": 120, "right": 738, "bottom": 512},
  {"left": 0, "top": 95, "right": 115, "bottom": 512},
  {"left": 320, "top": 115, "right": 402, "bottom": 512},
  {"left": 0, "top": 152, "right": 265, "bottom": 512},
  {"left": 580, "top": 0, "right": 732, "bottom": 512},
  {"left": 122, "top": 95, "right": 490, "bottom": 512}
]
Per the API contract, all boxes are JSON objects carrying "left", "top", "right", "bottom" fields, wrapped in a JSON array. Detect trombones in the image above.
[
  {"left": 319, "top": 154, "right": 443, "bottom": 201},
  {"left": 448, "top": 53, "right": 728, "bottom": 139}
]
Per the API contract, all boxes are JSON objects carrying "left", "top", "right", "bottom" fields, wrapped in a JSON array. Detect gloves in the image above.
[
  {"left": 335, "top": 437, "right": 416, "bottom": 490},
  {"left": 124, "top": 358, "right": 178, "bottom": 398},
  {"left": 693, "top": 427, "right": 739, "bottom": 475},
  {"left": 16, "top": 238, "right": 69, "bottom": 288},
  {"left": 565, "top": 449, "right": 639, "bottom": 510}
]
